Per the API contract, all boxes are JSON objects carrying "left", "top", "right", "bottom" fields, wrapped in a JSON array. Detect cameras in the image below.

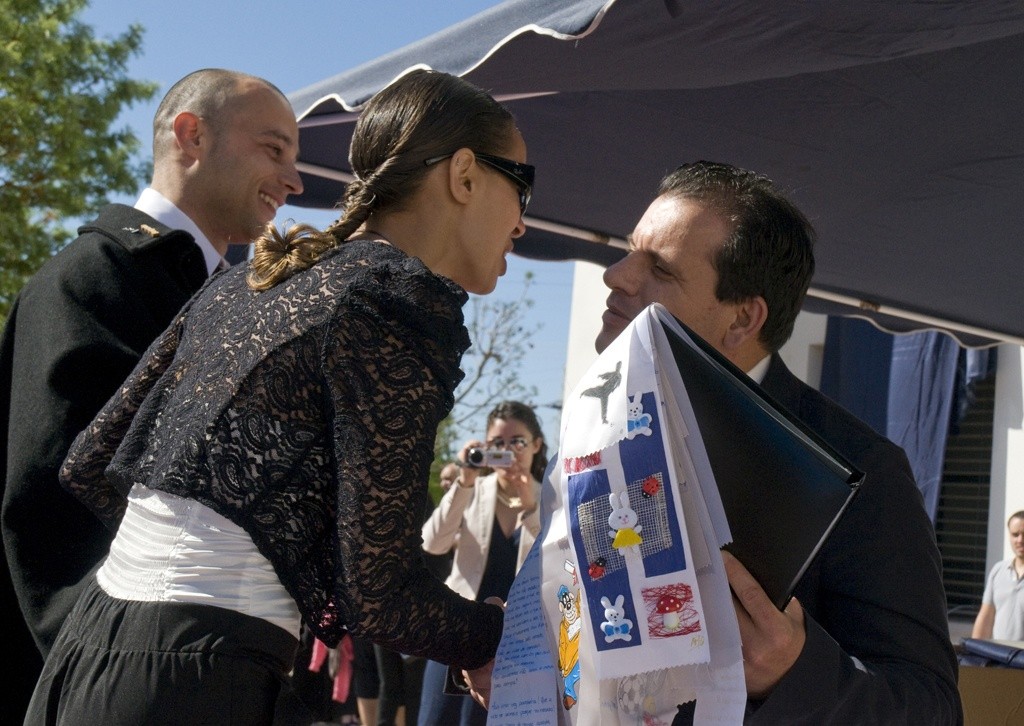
[{"left": 467, "top": 447, "right": 515, "bottom": 468}]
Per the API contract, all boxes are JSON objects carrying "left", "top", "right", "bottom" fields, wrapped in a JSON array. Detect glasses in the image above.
[
  {"left": 424, "top": 149, "right": 534, "bottom": 214},
  {"left": 496, "top": 436, "right": 535, "bottom": 455}
]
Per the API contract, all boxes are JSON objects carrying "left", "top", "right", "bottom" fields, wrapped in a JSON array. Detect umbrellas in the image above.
[{"left": 285, "top": 0, "right": 1024, "bottom": 348}]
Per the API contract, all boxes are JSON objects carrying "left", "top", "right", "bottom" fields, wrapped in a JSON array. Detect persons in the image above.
[
  {"left": 972, "top": 510, "right": 1024, "bottom": 641},
  {"left": 463, "top": 159, "right": 964, "bottom": 726},
  {"left": 22, "top": 69, "right": 534, "bottom": 726},
  {"left": 274, "top": 399, "right": 549, "bottom": 726},
  {"left": 0, "top": 69, "right": 304, "bottom": 726}
]
